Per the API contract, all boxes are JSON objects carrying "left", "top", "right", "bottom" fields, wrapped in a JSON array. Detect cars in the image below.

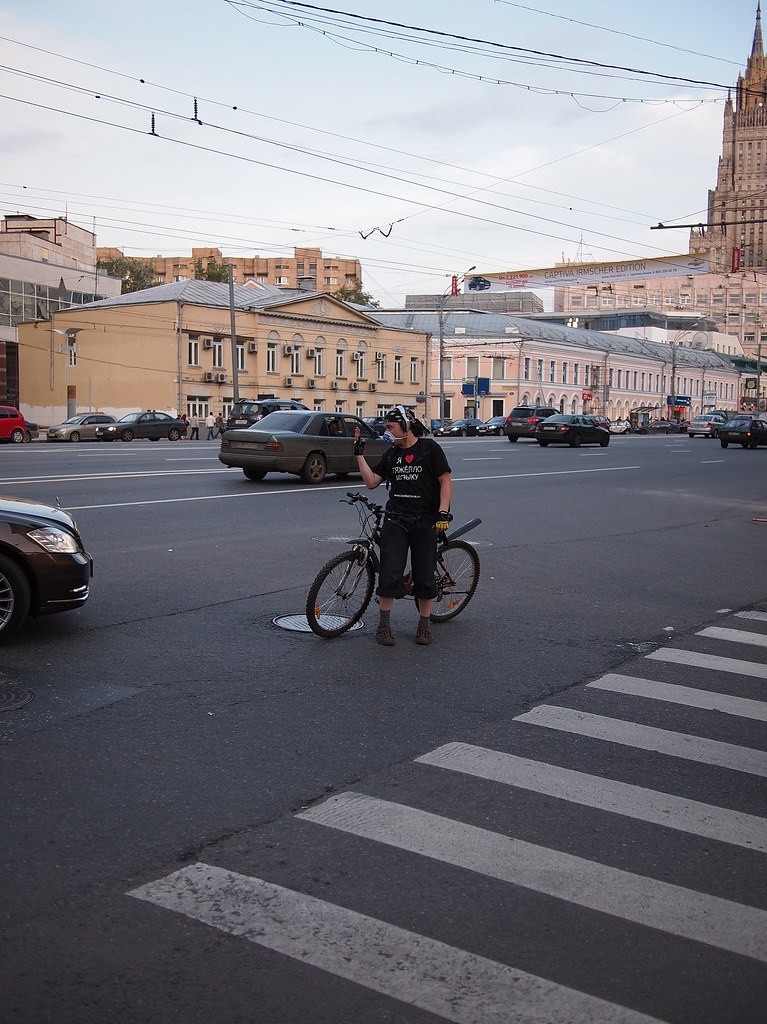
[
  {"left": 534, "top": 414, "right": 610, "bottom": 449},
  {"left": 0, "top": 494, "right": 94, "bottom": 647},
  {"left": 717, "top": 419, "right": 767, "bottom": 450},
  {"left": 0, "top": 405, "right": 26, "bottom": 444},
  {"left": 588, "top": 415, "right": 611, "bottom": 431},
  {"left": 218, "top": 409, "right": 392, "bottom": 486},
  {"left": 23, "top": 420, "right": 39, "bottom": 443},
  {"left": 609, "top": 420, "right": 690, "bottom": 435},
  {"left": 687, "top": 415, "right": 728, "bottom": 439},
  {"left": 94, "top": 412, "right": 187, "bottom": 442},
  {"left": 431, "top": 419, "right": 483, "bottom": 437},
  {"left": 46, "top": 414, "right": 119, "bottom": 443},
  {"left": 475, "top": 416, "right": 507, "bottom": 437},
  {"left": 359, "top": 417, "right": 386, "bottom": 437}
]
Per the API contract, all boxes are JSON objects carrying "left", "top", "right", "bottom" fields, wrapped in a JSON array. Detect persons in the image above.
[
  {"left": 583, "top": 412, "right": 686, "bottom": 430},
  {"left": 331, "top": 419, "right": 343, "bottom": 436},
  {"left": 419, "top": 414, "right": 428, "bottom": 438},
  {"left": 147, "top": 409, "right": 225, "bottom": 440},
  {"left": 353, "top": 405, "right": 452, "bottom": 645}
]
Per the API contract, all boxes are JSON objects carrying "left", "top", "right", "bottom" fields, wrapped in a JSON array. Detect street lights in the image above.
[
  {"left": 671, "top": 322, "right": 698, "bottom": 419},
  {"left": 440, "top": 266, "right": 477, "bottom": 428}
]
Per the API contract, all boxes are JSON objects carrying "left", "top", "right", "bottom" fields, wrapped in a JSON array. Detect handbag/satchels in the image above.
[{"left": 221, "top": 428, "right": 225, "bottom": 434}]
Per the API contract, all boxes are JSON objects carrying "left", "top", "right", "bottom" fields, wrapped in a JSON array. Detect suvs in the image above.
[
  {"left": 504, "top": 405, "right": 563, "bottom": 442},
  {"left": 226, "top": 399, "right": 310, "bottom": 431}
]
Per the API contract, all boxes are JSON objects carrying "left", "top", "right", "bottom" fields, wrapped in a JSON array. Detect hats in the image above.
[{"left": 385, "top": 408, "right": 418, "bottom": 424}]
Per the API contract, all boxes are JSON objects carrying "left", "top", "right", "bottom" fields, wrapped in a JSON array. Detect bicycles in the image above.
[{"left": 305, "top": 491, "right": 482, "bottom": 640}]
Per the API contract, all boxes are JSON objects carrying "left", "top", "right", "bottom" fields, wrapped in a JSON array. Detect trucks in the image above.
[{"left": 706, "top": 410, "right": 767, "bottom": 426}]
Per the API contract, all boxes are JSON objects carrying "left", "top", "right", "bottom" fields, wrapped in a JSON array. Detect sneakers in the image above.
[
  {"left": 376, "top": 623, "right": 396, "bottom": 645},
  {"left": 416, "top": 627, "right": 432, "bottom": 644}
]
